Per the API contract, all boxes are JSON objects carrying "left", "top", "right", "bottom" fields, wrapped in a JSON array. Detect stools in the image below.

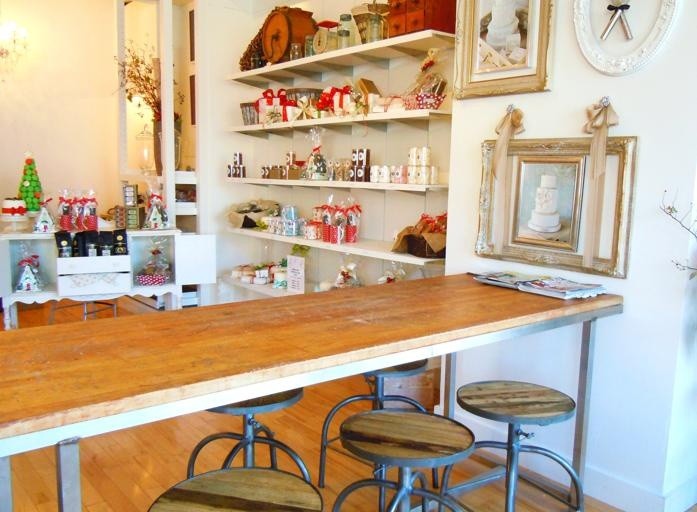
[
  {"left": 188, "top": 389, "right": 312, "bottom": 480},
  {"left": 318, "top": 357, "right": 427, "bottom": 512},
  {"left": 432, "top": 379, "right": 583, "bottom": 512},
  {"left": 331, "top": 409, "right": 475, "bottom": 512},
  {"left": 148, "top": 466, "right": 323, "bottom": 512}
]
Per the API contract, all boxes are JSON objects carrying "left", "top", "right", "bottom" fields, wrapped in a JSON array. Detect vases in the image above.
[{"left": 152, "top": 119, "right": 180, "bottom": 176}]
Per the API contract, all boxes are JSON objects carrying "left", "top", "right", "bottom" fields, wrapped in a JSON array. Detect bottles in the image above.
[
  {"left": 289, "top": 36, "right": 316, "bottom": 59},
  {"left": 250, "top": 48, "right": 261, "bottom": 70},
  {"left": 337, "top": 14, "right": 355, "bottom": 49},
  {"left": 365, "top": 14, "right": 390, "bottom": 43}
]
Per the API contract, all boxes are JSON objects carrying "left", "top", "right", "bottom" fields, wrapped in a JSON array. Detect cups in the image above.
[
  {"left": 260, "top": 205, "right": 360, "bottom": 244},
  {"left": 158, "top": 126, "right": 182, "bottom": 171},
  {"left": 132, "top": 124, "right": 157, "bottom": 176},
  {"left": 55, "top": 202, "right": 96, "bottom": 231}
]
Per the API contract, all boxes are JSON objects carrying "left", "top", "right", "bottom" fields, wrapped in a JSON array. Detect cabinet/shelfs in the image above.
[{"left": 216, "top": 30, "right": 455, "bottom": 297}]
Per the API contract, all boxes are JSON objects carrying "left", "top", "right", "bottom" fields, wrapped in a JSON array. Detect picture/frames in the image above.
[
  {"left": 476, "top": 136, "right": 638, "bottom": 278},
  {"left": 448, "top": 1, "right": 550, "bottom": 101}
]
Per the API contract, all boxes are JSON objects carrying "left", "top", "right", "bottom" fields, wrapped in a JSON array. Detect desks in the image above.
[
  {"left": 0, "top": 272, "right": 622, "bottom": 512},
  {"left": 1, "top": 229, "right": 177, "bottom": 330}
]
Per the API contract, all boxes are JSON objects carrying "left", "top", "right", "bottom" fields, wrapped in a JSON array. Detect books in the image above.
[{"left": 473, "top": 268, "right": 605, "bottom": 300}]
